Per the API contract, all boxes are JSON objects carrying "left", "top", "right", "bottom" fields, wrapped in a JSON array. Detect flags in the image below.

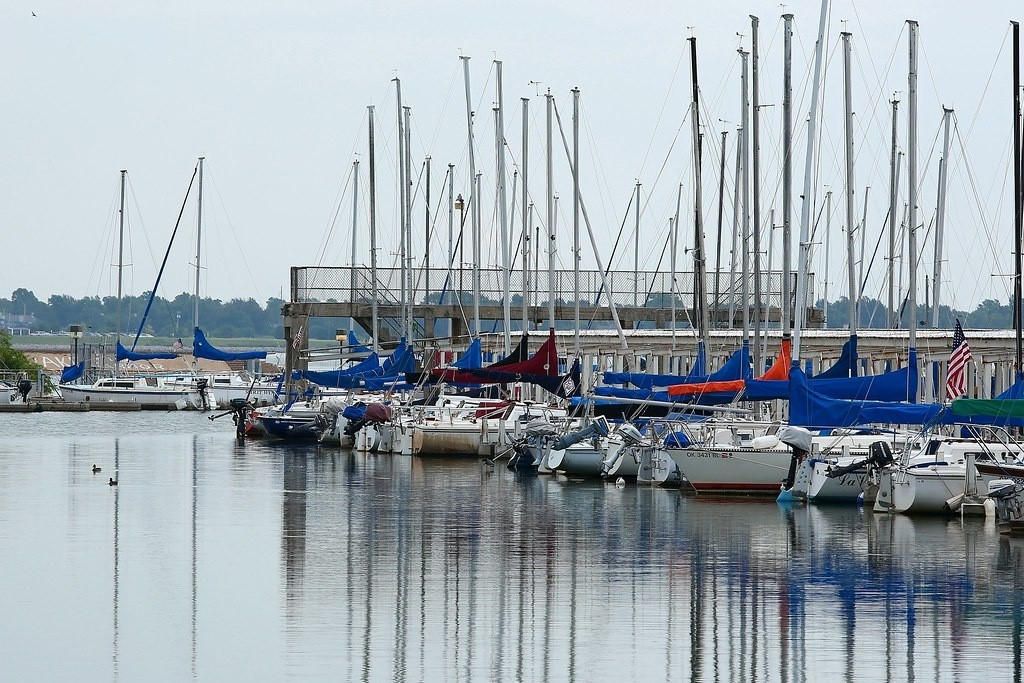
[
  {"left": 173, "top": 339, "right": 183, "bottom": 349},
  {"left": 945, "top": 317, "right": 974, "bottom": 398},
  {"left": 292, "top": 325, "right": 305, "bottom": 350}
]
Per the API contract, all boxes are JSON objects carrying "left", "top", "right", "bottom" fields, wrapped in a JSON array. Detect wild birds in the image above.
[
  {"left": 109, "top": 478, "right": 118, "bottom": 487},
  {"left": 92, "top": 464, "right": 101, "bottom": 472}
]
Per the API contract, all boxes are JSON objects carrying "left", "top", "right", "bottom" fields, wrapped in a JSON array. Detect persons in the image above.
[{"left": 295, "top": 384, "right": 323, "bottom": 407}]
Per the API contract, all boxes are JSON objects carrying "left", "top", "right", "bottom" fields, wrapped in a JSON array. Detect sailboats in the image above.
[{"left": 0, "top": 1, "right": 1024, "bottom": 534}]
